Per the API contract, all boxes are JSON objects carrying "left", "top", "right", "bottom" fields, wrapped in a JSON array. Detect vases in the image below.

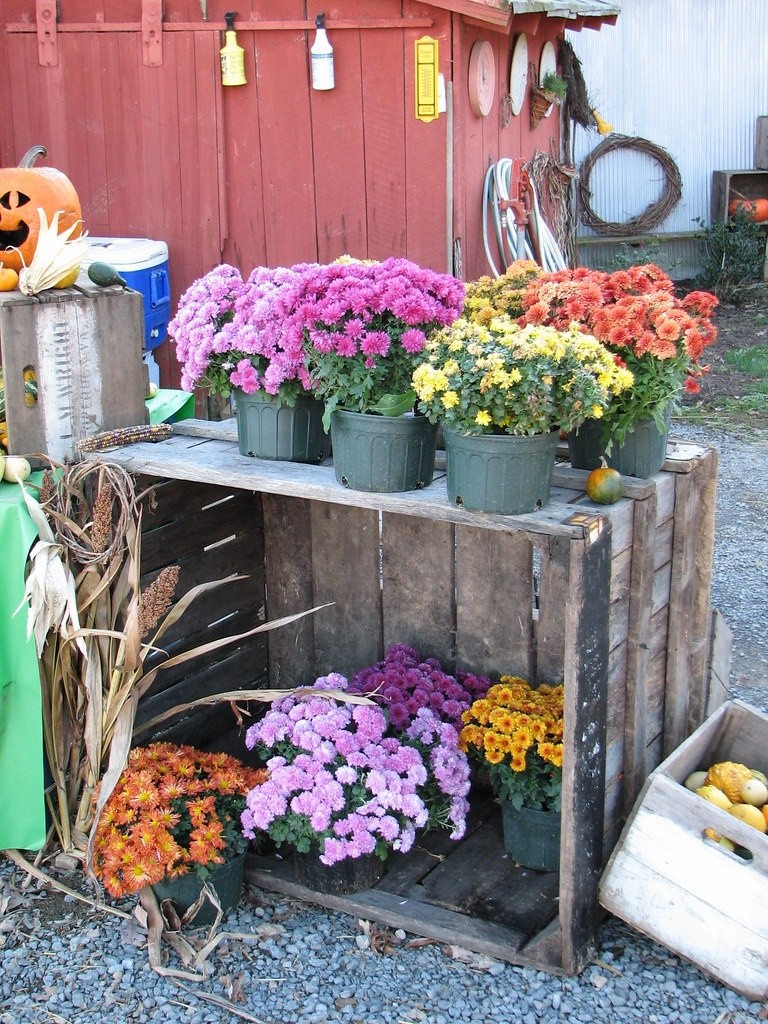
[
  {"left": 501, "top": 800, "right": 561, "bottom": 872},
  {"left": 221, "top": 389, "right": 670, "bottom": 515},
  {"left": 151, "top": 848, "right": 248, "bottom": 928},
  {"left": 292, "top": 837, "right": 383, "bottom": 895}
]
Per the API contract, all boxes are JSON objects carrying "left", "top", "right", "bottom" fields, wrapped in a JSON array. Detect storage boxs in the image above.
[
  {"left": 0, "top": 236, "right": 172, "bottom": 469},
  {"left": 80, "top": 418, "right": 718, "bottom": 978},
  {"left": 598, "top": 699, "right": 768, "bottom": 1004},
  {"left": 711, "top": 116, "right": 768, "bottom": 232}
]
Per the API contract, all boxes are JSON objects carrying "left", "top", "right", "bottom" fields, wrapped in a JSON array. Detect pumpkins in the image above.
[
  {"left": 88, "top": 262, "right": 127, "bottom": 286},
  {"left": 729, "top": 198, "right": 768, "bottom": 222},
  {"left": 0, "top": 268, "right": 18, "bottom": 291},
  {"left": 0, "top": 365, "right": 38, "bottom": 485},
  {"left": 685, "top": 761, "right": 768, "bottom": 853},
  {"left": 53, "top": 265, "right": 80, "bottom": 288},
  {"left": 586, "top": 455, "right": 623, "bottom": 505}
]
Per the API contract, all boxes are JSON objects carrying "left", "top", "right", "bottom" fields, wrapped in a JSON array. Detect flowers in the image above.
[
  {"left": 86, "top": 642, "right": 566, "bottom": 896},
  {"left": 166, "top": 256, "right": 719, "bottom": 457}
]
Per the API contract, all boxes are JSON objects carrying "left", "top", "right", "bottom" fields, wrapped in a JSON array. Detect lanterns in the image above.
[{"left": 0, "top": 164, "right": 82, "bottom": 276}]
